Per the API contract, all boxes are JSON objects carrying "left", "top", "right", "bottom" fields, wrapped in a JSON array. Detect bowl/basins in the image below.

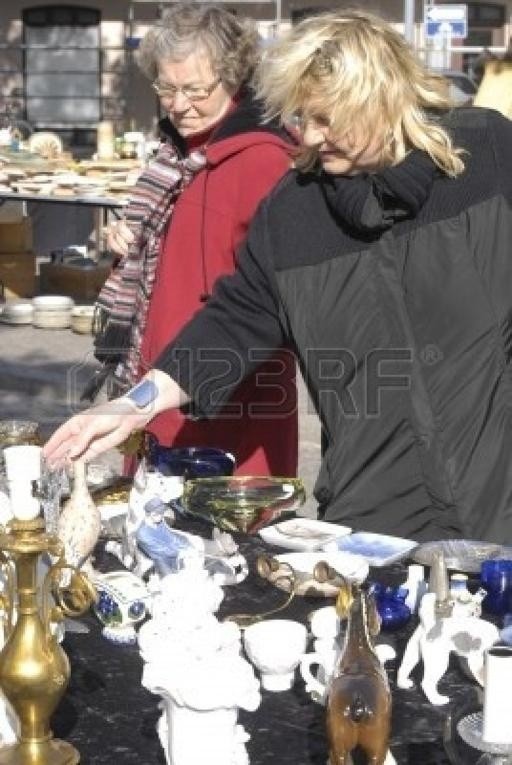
[
  {"left": 71, "top": 305, "right": 103, "bottom": 334},
  {"left": 154, "top": 446, "right": 235, "bottom": 477}
]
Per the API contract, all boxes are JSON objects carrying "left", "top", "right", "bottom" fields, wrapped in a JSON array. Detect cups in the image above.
[
  {"left": 372, "top": 560, "right": 512, "bottom": 634},
  {"left": 481, "top": 646, "right": 512, "bottom": 741},
  {"left": 2, "top": 444, "right": 43, "bottom": 522},
  {"left": 96, "top": 122, "right": 114, "bottom": 162},
  {"left": 242, "top": 619, "right": 307, "bottom": 692}
]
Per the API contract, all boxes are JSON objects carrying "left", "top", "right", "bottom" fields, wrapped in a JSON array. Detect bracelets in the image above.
[{"left": 127, "top": 380, "right": 160, "bottom": 408}]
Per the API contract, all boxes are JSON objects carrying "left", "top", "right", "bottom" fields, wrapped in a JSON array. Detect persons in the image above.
[
  {"left": 42, "top": 7, "right": 512, "bottom": 547},
  {"left": 474, "top": 34, "right": 512, "bottom": 120},
  {"left": 90, "top": 2, "right": 299, "bottom": 535}
]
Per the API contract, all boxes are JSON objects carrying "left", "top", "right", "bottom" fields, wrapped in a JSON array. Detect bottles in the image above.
[
  {"left": 120, "top": 141, "right": 137, "bottom": 159},
  {"left": 0, "top": 419, "right": 41, "bottom": 496}
]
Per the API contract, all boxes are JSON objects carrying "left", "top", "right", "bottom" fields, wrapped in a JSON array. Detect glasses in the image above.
[{"left": 150, "top": 75, "right": 220, "bottom": 101}]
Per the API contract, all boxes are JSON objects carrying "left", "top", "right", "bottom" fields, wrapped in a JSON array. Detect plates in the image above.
[
  {"left": 258, "top": 517, "right": 511, "bottom": 598},
  {"left": 11, "top": 172, "right": 110, "bottom": 202},
  {"left": 95, "top": 501, "right": 175, "bottom": 540},
  {"left": 0, "top": 294, "right": 74, "bottom": 331}
]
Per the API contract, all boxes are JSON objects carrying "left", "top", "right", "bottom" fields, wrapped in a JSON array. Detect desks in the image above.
[
  {"left": 1, "top": 156, "right": 148, "bottom": 259},
  {"left": 0, "top": 477, "right": 512, "bottom": 765}
]
